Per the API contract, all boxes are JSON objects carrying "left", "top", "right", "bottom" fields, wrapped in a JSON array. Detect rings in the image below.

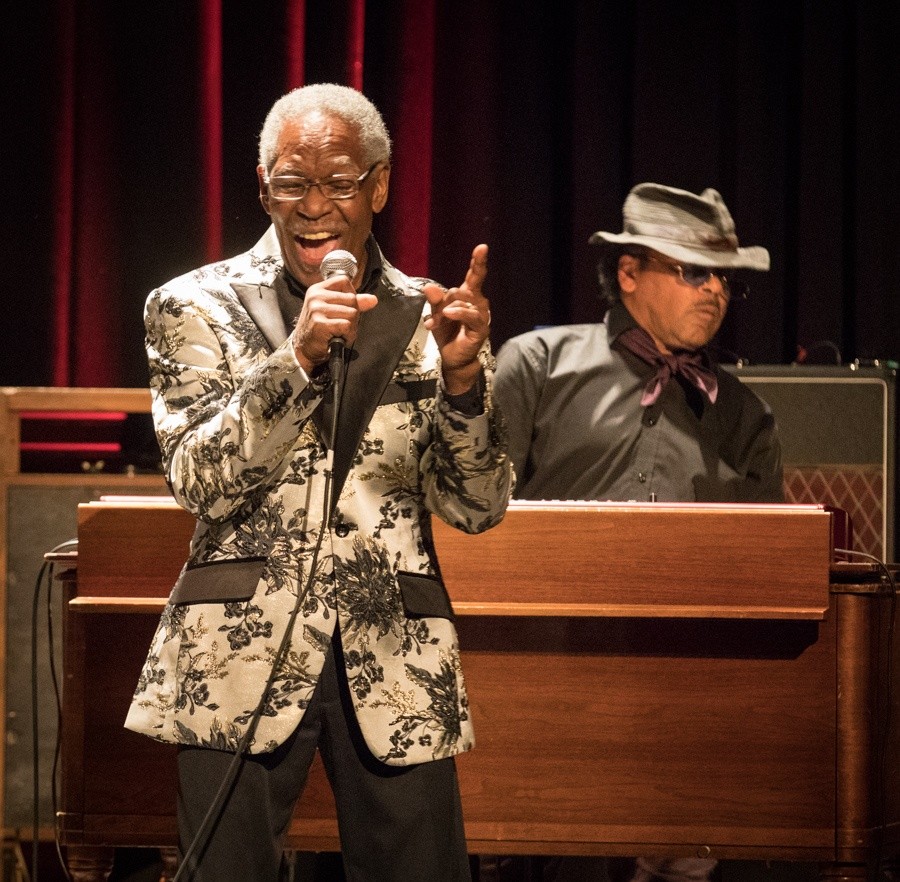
[{"left": 486, "top": 309, "right": 492, "bottom": 325}]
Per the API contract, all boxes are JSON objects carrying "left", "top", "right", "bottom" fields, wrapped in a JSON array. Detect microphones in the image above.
[{"left": 316, "top": 250, "right": 358, "bottom": 383}]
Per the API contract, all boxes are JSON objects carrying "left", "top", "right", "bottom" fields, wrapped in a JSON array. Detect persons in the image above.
[
  {"left": 479, "top": 185, "right": 789, "bottom": 882},
  {"left": 145, "top": 86, "right": 512, "bottom": 882}
]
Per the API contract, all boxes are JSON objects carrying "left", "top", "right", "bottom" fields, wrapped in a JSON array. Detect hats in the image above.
[{"left": 588, "top": 182, "right": 772, "bottom": 271}]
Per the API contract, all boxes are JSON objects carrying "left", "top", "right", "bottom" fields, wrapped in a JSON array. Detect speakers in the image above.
[{"left": 717, "top": 360, "right": 900, "bottom": 579}]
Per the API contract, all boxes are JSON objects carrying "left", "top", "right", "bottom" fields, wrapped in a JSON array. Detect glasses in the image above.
[
  {"left": 643, "top": 255, "right": 737, "bottom": 290},
  {"left": 262, "top": 159, "right": 381, "bottom": 200}
]
